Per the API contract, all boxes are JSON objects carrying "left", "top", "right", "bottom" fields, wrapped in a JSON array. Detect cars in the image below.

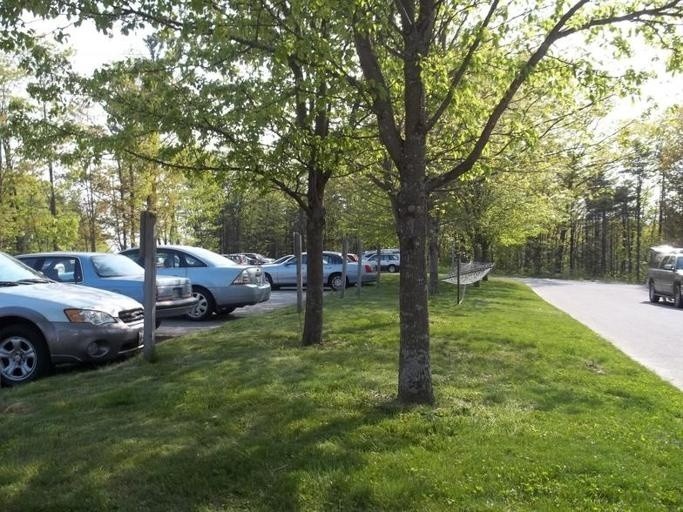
[
  {"left": 0, "top": 251, "right": 146, "bottom": 388},
  {"left": 12, "top": 252, "right": 197, "bottom": 330},
  {"left": 220, "top": 245, "right": 399, "bottom": 293},
  {"left": 116, "top": 245, "right": 272, "bottom": 319}
]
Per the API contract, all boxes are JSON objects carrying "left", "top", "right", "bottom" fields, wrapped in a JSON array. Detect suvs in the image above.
[{"left": 647, "top": 244, "right": 683, "bottom": 310}]
